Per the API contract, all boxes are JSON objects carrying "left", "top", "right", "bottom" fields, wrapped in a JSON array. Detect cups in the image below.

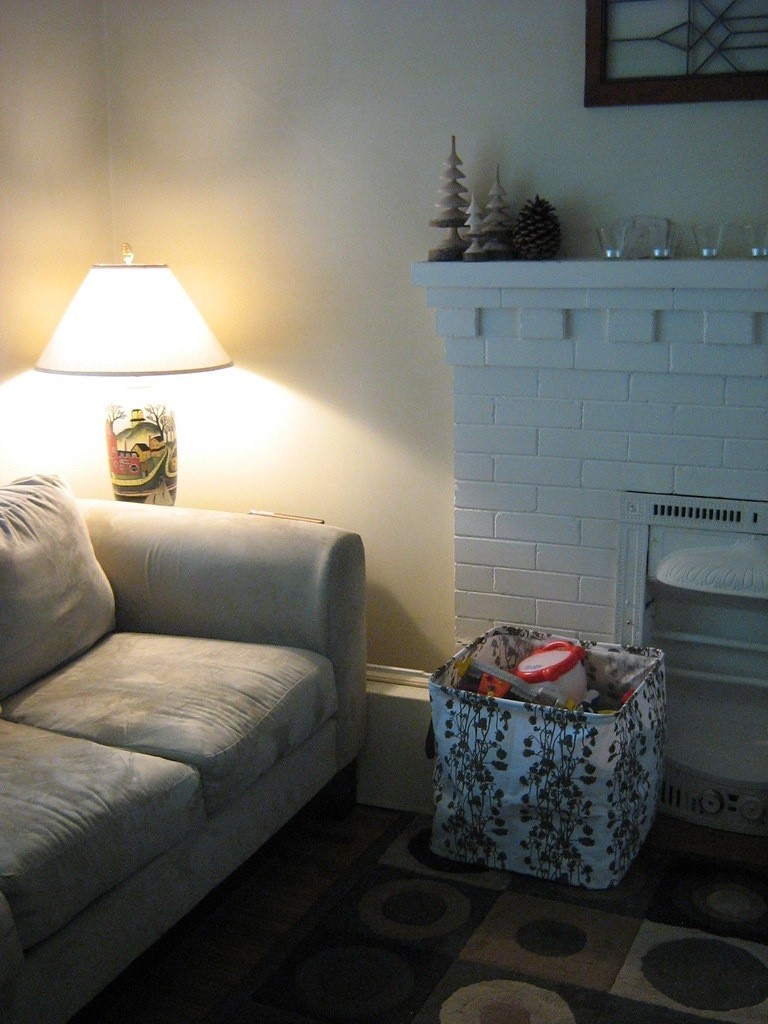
[
  {"left": 692, "top": 224, "right": 726, "bottom": 257},
  {"left": 646, "top": 224, "right": 679, "bottom": 259},
  {"left": 743, "top": 223, "right": 768, "bottom": 258},
  {"left": 596, "top": 225, "right": 628, "bottom": 258}
]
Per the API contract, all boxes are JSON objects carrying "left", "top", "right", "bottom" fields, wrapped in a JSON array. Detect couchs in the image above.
[{"left": 1, "top": 477, "right": 366, "bottom": 1022}]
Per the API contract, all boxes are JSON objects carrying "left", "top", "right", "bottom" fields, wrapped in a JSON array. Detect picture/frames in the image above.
[{"left": 582, "top": 1, "right": 767, "bottom": 109}]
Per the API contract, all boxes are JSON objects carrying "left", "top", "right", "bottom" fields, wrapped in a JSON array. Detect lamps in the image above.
[{"left": 36, "top": 244, "right": 236, "bottom": 507}]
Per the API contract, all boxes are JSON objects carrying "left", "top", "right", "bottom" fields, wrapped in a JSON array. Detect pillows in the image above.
[{"left": 1, "top": 464, "right": 122, "bottom": 712}]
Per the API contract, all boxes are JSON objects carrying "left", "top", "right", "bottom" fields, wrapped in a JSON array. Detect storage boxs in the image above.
[{"left": 427, "top": 627, "right": 667, "bottom": 887}]
